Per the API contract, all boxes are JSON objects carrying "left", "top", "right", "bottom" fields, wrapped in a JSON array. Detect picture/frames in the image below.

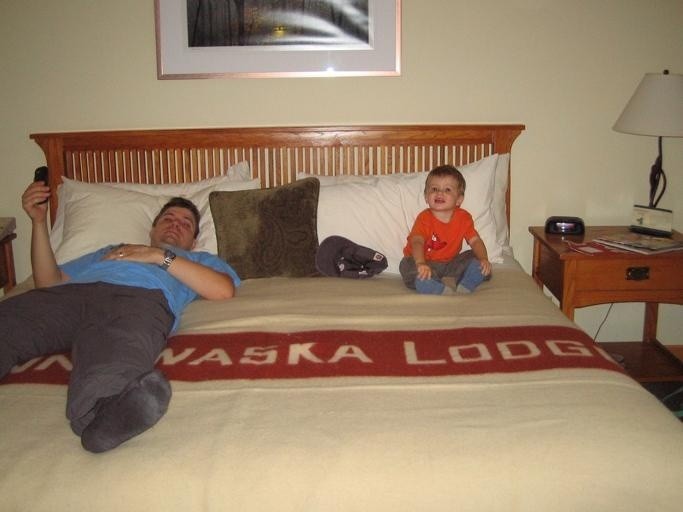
[{"left": 154, "top": 0, "right": 402, "bottom": 80}]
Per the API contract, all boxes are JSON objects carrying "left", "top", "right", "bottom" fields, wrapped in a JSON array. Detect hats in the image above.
[{"left": 315, "top": 235, "right": 389, "bottom": 281}]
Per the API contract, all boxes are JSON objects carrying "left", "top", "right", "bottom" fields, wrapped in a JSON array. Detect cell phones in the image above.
[
  {"left": 34, "top": 166, "right": 48, "bottom": 204},
  {"left": 571, "top": 243, "right": 603, "bottom": 257}
]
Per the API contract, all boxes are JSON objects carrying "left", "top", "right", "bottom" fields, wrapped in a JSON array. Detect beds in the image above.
[{"left": 0, "top": 125, "right": 683, "bottom": 512}]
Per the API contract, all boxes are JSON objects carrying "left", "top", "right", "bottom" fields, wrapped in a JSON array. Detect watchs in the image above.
[{"left": 160, "top": 249, "right": 175, "bottom": 270}]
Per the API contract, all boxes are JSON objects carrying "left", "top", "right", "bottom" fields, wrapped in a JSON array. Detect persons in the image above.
[
  {"left": 398, "top": 164, "right": 494, "bottom": 296},
  {"left": 0, "top": 177, "right": 243, "bottom": 454}
]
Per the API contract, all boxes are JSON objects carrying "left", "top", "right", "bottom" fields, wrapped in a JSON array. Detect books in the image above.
[{"left": 592, "top": 231, "right": 682, "bottom": 255}]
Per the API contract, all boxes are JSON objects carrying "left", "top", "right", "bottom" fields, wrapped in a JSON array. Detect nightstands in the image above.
[
  {"left": 0, "top": 233, "right": 17, "bottom": 296},
  {"left": 528, "top": 226, "right": 683, "bottom": 383}
]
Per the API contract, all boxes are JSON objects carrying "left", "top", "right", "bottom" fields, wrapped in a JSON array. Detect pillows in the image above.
[
  {"left": 49, "top": 160, "right": 251, "bottom": 255},
  {"left": 209, "top": 177, "right": 320, "bottom": 281},
  {"left": 315, "top": 153, "right": 504, "bottom": 274},
  {"left": 56, "top": 173, "right": 262, "bottom": 266},
  {"left": 297, "top": 152, "right": 509, "bottom": 256}
]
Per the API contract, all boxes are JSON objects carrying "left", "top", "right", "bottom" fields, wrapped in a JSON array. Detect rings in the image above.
[{"left": 119, "top": 252, "right": 123, "bottom": 258}]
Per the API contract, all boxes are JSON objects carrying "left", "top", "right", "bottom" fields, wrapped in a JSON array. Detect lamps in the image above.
[{"left": 613, "top": 70, "right": 683, "bottom": 237}]
